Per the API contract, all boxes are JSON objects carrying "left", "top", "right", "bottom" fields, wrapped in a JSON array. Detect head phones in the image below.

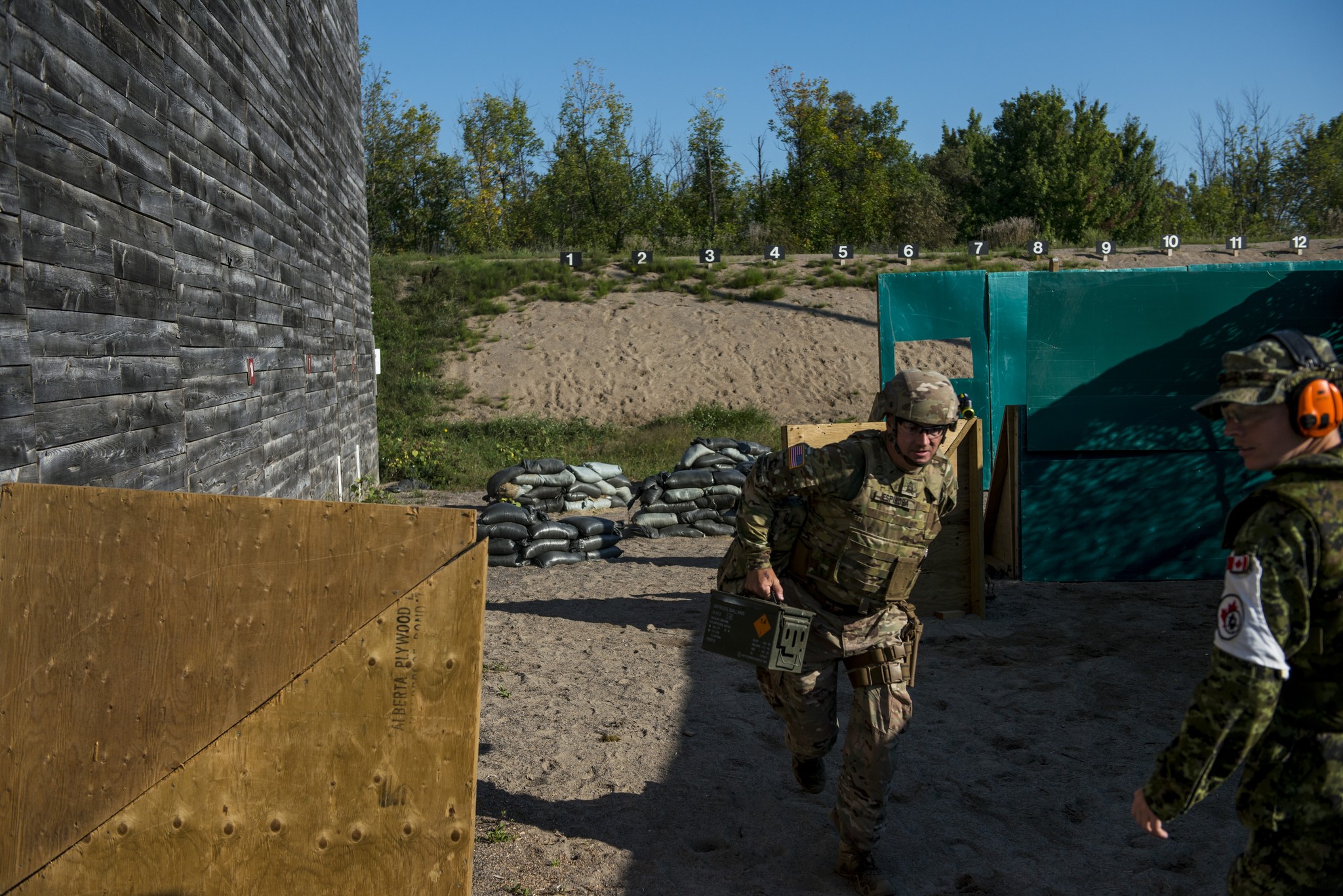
[{"left": 1258, "top": 327, "right": 1343, "bottom": 438}]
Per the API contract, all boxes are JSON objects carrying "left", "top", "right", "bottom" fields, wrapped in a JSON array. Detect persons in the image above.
[
  {"left": 717, "top": 370, "right": 959, "bottom": 896},
  {"left": 1130, "top": 330, "right": 1343, "bottom": 896}
]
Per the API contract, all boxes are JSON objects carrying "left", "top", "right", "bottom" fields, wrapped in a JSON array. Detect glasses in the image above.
[
  {"left": 902, "top": 421, "right": 949, "bottom": 436},
  {"left": 1219, "top": 404, "right": 1265, "bottom": 422}
]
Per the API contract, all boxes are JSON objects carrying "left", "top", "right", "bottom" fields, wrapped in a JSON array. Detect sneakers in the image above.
[
  {"left": 834, "top": 852, "right": 895, "bottom": 896},
  {"left": 792, "top": 755, "right": 826, "bottom": 794}
]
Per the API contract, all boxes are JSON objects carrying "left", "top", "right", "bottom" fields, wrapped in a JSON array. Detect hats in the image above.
[
  {"left": 873, "top": 371, "right": 961, "bottom": 432},
  {"left": 1190, "top": 328, "right": 1343, "bottom": 421}
]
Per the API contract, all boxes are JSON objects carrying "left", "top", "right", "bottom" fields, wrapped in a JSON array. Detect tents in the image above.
[{"left": 875, "top": 262, "right": 1343, "bottom": 612}]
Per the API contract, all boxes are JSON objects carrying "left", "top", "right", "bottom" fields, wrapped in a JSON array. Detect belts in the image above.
[{"left": 795, "top": 578, "right": 873, "bottom": 616}]
